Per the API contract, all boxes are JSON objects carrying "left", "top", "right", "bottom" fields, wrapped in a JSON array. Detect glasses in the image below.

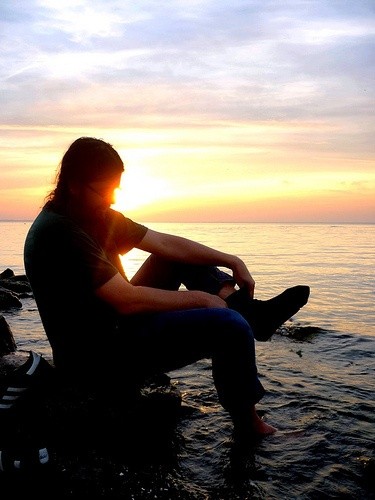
[{"left": 84, "top": 180, "right": 122, "bottom": 197}]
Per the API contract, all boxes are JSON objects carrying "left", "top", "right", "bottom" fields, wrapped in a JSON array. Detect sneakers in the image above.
[{"left": 0, "top": 349, "right": 49, "bottom": 432}]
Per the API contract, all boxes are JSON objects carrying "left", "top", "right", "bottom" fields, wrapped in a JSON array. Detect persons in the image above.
[{"left": 23, "top": 137, "right": 311, "bottom": 436}]
